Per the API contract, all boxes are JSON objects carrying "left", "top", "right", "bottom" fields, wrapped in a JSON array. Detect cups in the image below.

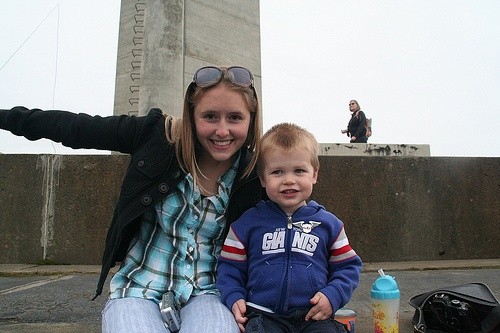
[{"left": 334, "top": 310, "right": 356, "bottom": 333}]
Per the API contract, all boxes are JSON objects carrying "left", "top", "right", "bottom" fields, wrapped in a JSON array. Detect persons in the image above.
[
  {"left": 340, "top": 100, "right": 368, "bottom": 143},
  {"left": 0, "top": 66, "right": 260, "bottom": 333},
  {"left": 215, "top": 123, "right": 362, "bottom": 333}
]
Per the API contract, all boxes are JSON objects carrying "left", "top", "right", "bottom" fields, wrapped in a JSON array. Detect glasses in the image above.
[
  {"left": 349, "top": 103, "right": 354, "bottom": 106},
  {"left": 192, "top": 67, "right": 254, "bottom": 88}
]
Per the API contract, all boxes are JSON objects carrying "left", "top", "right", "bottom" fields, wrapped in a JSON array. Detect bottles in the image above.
[{"left": 370, "top": 269, "right": 400, "bottom": 333}]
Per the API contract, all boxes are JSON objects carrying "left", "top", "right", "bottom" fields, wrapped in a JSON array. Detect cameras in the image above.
[
  {"left": 422, "top": 292, "right": 477, "bottom": 333},
  {"left": 160, "top": 292, "right": 182, "bottom": 332}
]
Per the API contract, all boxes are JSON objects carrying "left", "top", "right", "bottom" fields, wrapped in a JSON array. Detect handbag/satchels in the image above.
[{"left": 408, "top": 282, "right": 499, "bottom": 333}]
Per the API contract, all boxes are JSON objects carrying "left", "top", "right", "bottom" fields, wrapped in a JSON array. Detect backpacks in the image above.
[{"left": 365, "top": 119, "right": 372, "bottom": 137}]
